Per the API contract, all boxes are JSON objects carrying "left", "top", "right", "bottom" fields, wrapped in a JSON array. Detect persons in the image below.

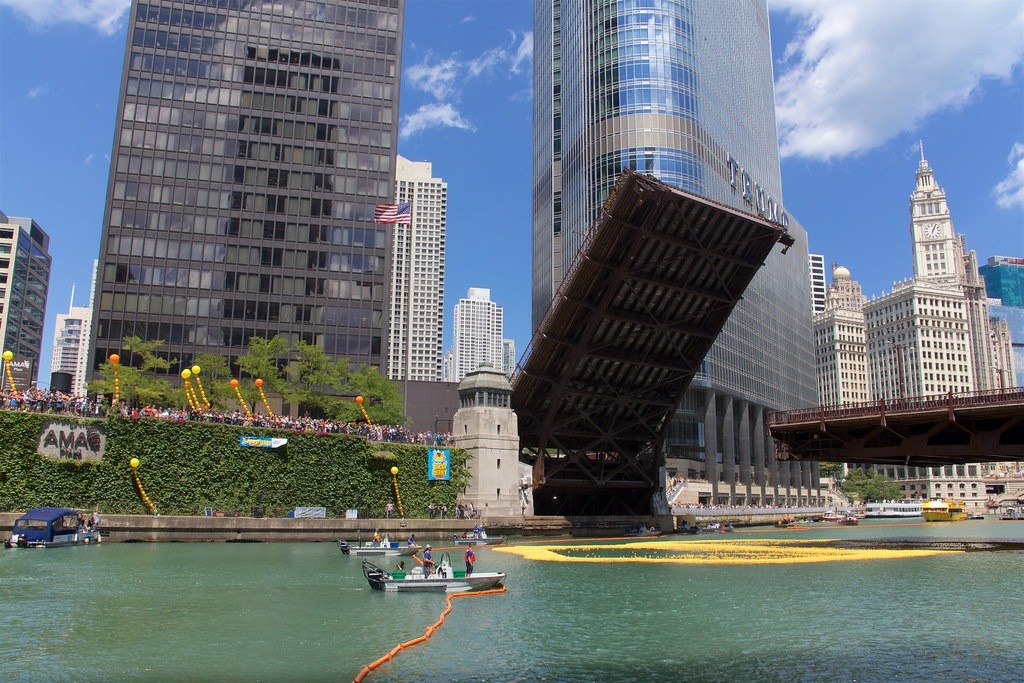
[
  {"left": 422, "top": 544, "right": 435, "bottom": 579},
  {"left": 466, "top": 543, "right": 475, "bottom": 578},
  {"left": 2, "top": 388, "right": 456, "bottom": 448},
  {"left": 407, "top": 534, "right": 418, "bottom": 547}
]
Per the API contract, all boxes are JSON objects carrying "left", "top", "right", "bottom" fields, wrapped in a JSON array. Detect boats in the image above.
[
  {"left": 708, "top": 523, "right": 720, "bottom": 530},
  {"left": 921, "top": 500, "right": 968, "bottom": 521},
  {"left": 773, "top": 523, "right": 795, "bottom": 527},
  {"left": 972, "top": 514, "right": 984, "bottom": 519},
  {"left": 3, "top": 507, "right": 101, "bottom": 548},
  {"left": 999, "top": 513, "right": 1013, "bottom": 519},
  {"left": 336, "top": 533, "right": 422, "bottom": 556},
  {"left": 453, "top": 535, "right": 504, "bottom": 546},
  {"left": 719, "top": 525, "right": 734, "bottom": 531},
  {"left": 820, "top": 512, "right": 846, "bottom": 522},
  {"left": 624, "top": 527, "right": 662, "bottom": 537},
  {"left": 361, "top": 552, "right": 507, "bottom": 593},
  {"left": 841, "top": 517, "right": 858, "bottom": 525},
  {"left": 673, "top": 528, "right": 697, "bottom": 534}
]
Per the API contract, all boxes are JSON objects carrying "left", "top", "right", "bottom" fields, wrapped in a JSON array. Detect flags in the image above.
[{"left": 374, "top": 202, "right": 412, "bottom": 226}]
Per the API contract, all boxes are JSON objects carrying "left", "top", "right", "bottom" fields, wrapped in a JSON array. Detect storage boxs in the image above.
[
  {"left": 454, "top": 570, "right": 465, "bottom": 579},
  {"left": 391, "top": 570, "right": 406, "bottom": 579},
  {"left": 346, "top": 510, "right": 358, "bottom": 518},
  {"left": 390, "top": 543, "right": 399, "bottom": 548}
]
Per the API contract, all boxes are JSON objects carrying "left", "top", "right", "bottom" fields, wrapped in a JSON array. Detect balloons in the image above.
[
  {"left": 129, "top": 457, "right": 158, "bottom": 514},
  {"left": 110, "top": 353, "right": 120, "bottom": 404},
  {"left": 254, "top": 379, "right": 273, "bottom": 414},
  {"left": 230, "top": 379, "right": 251, "bottom": 417},
  {"left": 181, "top": 365, "right": 210, "bottom": 412},
  {"left": 1, "top": 349, "right": 24, "bottom": 409}
]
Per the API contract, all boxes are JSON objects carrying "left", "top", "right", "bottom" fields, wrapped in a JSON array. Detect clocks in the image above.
[{"left": 924, "top": 223, "right": 942, "bottom": 240}]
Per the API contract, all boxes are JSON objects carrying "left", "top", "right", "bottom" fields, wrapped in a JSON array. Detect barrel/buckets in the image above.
[
  {"left": 391, "top": 572, "right": 406, "bottom": 579},
  {"left": 453, "top": 570, "right": 467, "bottom": 578}
]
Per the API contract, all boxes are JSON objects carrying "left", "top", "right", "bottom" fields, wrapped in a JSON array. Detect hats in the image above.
[{"left": 425, "top": 544, "right": 431, "bottom": 548}]
